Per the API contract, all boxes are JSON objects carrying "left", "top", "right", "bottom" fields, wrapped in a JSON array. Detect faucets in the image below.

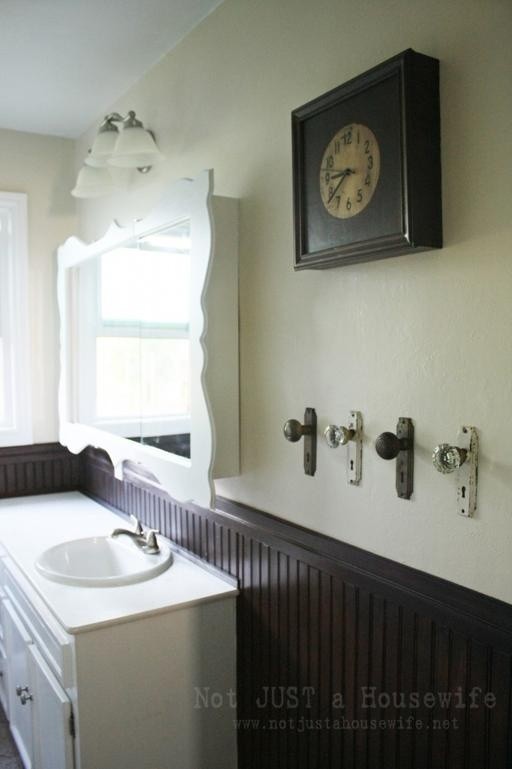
[{"left": 111, "top": 528, "right": 148, "bottom": 548}]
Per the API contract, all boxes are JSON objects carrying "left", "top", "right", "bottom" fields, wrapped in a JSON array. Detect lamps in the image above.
[{"left": 71, "top": 109, "right": 161, "bottom": 200}]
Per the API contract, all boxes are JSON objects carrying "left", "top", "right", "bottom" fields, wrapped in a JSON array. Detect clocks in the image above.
[{"left": 288, "top": 46, "right": 439, "bottom": 271}]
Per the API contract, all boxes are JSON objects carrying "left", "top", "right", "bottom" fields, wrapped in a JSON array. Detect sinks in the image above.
[{"left": 35, "top": 536, "right": 174, "bottom": 588}]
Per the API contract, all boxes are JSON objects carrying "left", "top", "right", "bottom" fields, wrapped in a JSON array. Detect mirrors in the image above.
[{"left": 56, "top": 167, "right": 242, "bottom": 512}]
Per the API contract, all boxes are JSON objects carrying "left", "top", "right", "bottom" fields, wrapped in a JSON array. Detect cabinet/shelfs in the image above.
[{"left": 2, "top": 547, "right": 237, "bottom": 767}]
